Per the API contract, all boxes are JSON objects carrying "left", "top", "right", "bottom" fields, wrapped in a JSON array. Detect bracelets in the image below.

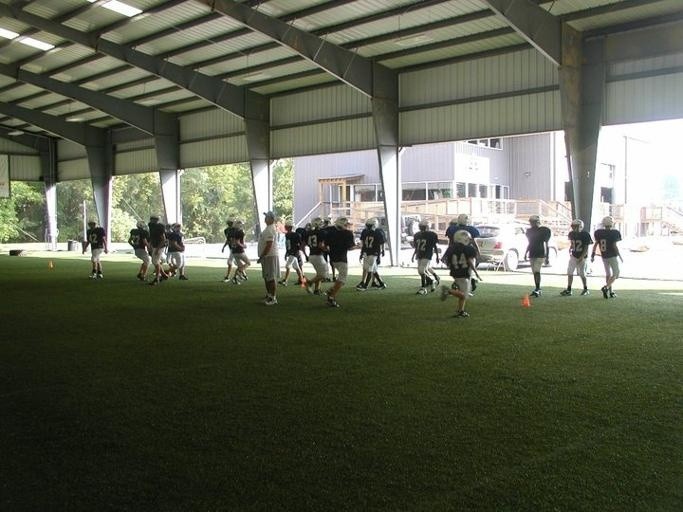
[{"left": 591, "top": 251, "right": 595, "bottom": 257}]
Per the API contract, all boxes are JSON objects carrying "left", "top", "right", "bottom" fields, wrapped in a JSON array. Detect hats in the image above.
[{"left": 264, "top": 211, "right": 275, "bottom": 218}]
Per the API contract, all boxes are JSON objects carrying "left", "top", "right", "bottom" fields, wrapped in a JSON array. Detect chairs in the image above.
[
  {"left": 432, "top": 248, "right": 443, "bottom": 268},
  {"left": 486, "top": 249, "right": 506, "bottom": 271}
]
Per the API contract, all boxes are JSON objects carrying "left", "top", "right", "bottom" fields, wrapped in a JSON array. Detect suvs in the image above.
[
  {"left": 355, "top": 213, "right": 424, "bottom": 249},
  {"left": 470, "top": 223, "right": 558, "bottom": 273}
]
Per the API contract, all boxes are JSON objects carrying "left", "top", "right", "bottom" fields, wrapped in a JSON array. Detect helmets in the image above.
[
  {"left": 602, "top": 216, "right": 616, "bottom": 226},
  {"left": 136, "top": 221, "right": 181, "bottom": 232},
  {"left": 150, "top": 215, "right": 161, "bottom": 224},
  {"left": 88, "top": 218, "right": 98, "bottom": 228},
  {"left": 451, "top": 214, "right": 472, "bottom": 246},
  {"left": 419, "top": 219, "right": 431, "bottom": 231},
  {"left": 529, "top": 215, "right": 540, "bottom": 228},
  {"left": 284, "top": 216, "right": 353, "bottom": 229},
  {"left": 226, "top": 217, "right": 244, "bottom": 229},
  {"left": 365, "top": 218, "right": 379, "bottom": 231},
  {"left": 571, "top": 219, "right": 584, "bottom": 232}
]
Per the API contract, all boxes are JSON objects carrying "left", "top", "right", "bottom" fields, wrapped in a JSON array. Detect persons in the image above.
[
  {"left": 127, "top": 215, "right": 188, "bottom": 286},
  {"left": 440, "top": 230, "right": 482, "bottom": 317},
  {"left": 81, "top": 219, "right": 107, "bottom": 279},
  {"left": 277, "top": 214, "right": 481, "bottom": 307},
  {"left": 523, "top": 215, "right": 550, "bottom": 295},
  {"left": 559, "top": 219, "right": 593, "bottom": 295},
  {"left": 256, "top": 211, "right": 277, "bottom": 305},
  {"left": 591, "top": 217, "right": 623, "bottom": 298}
]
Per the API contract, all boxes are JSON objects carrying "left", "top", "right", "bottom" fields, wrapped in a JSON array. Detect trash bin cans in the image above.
[{"left": 67, "top": 240, "right": 74, "bottom": 251}]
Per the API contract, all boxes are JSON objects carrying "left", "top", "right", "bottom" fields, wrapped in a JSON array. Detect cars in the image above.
[{"left": 470, "top": 217, "right": 571, "bottom": 252}]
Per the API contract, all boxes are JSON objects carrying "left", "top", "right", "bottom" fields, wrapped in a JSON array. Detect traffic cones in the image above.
[
  {"left": 522, "top": 292, "right": 532, "bottom": 307},
  {"left": 300, "top": 275, "right": 309, "bottom": 288},
  {"left": 48, "top": 259, "right": 54, "bottom": 270}
]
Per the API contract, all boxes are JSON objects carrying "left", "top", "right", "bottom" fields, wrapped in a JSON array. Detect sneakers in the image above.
[
  {"left": 278, "top": 279, "right": 287, "bottom": 286},
  {"left": 222, "top": 269, "right": 248, "bottom": 285},
  {"left": 300, "top": 276, "right": 337, "bottom": 295},
  {"left": 581, "top": 289, "right": 589, "bottom": 295},
  {"left": 610, "top": 292, "right": 617, "bottom": 297},
  {"left": 560, "top": 290, "right": 572, "bottom": 296},
  {"left": 601, "top": 286, "right": 609, "bottom": 299},
  {"left": 532, "top": 289, "right": 542, "bottom": 296},
  {"left": 416, "top": 276, "right": 440, "bottom": 295},
  {"left": 88, "top": 272, "right": 103, "bottom": 279},
  {"left": 261, "top": 293, "right": 278, "bottom": 305},
  {"left": 456, "top": 311, "right": 470, "bottom": 318},
  {"left": 324, "top": 296, "right": 340, "bottom": 308},
  {"left": 441, "top": 285, "right": 449, "bottom": 301},
  {"left": 356, "top": 281, "right": 387, "bottom": 291},
  {"left": 136, "top": 267, "right": 188, "bottom": 285}
]
